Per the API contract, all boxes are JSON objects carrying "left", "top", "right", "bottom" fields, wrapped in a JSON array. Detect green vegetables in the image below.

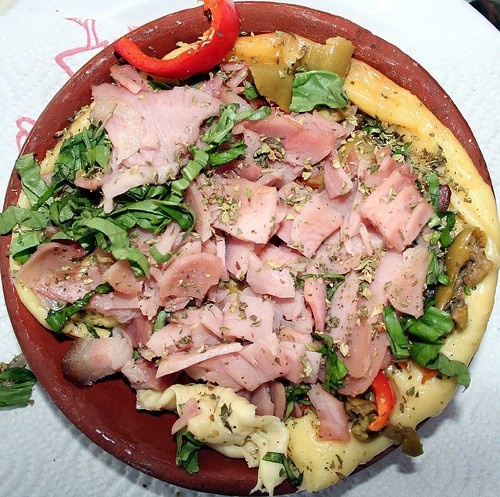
[{"left": 0, "top": 367, "right": 36, "bottom": 408}]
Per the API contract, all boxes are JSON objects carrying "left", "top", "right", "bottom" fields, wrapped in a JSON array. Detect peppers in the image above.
[{"left": 111, "top": 0, "right": 241, "bottom": 80}]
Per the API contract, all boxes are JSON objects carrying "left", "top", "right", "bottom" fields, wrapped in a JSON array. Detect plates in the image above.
[{"left": 0, "top": 2, "right": 500, "bottom": 495}]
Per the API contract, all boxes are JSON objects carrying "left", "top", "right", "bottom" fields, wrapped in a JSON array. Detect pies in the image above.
[{"left": 0, "top": 30, "right": 500, "bottom": 495}]
